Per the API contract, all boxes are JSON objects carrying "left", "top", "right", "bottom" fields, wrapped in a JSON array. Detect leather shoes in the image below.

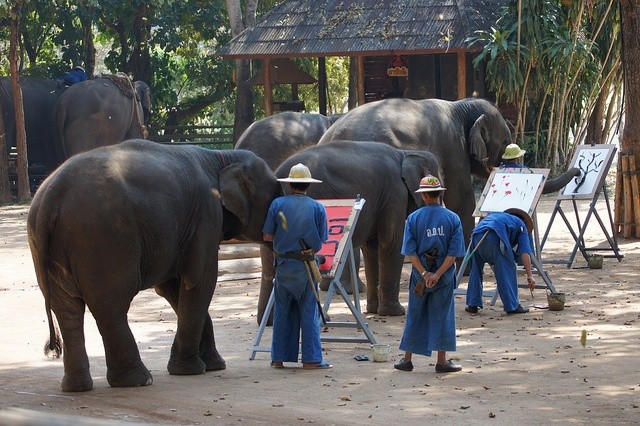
[
  {"left": 465, "top": 305, "right": 478, "bottom": 313},
  {"left": 394, "top": 358, "right": 413, "bottom": 371},
  {"left": 507, "top": 304, "right": 529, "bottom": 313},
  {"left": 436, "top": 358, "right": 462, "bottom": 372}
]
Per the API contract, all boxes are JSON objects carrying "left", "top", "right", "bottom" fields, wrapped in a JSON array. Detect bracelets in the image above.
[{"left": 421, "top": 271, "right": 427, "bottom": 276}]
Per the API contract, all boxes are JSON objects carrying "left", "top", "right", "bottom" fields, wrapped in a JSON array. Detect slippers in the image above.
[
  {"left": 271, "top": 361, "right": 284, "bottom": 368},
  {"left": 303, "top": 362, "right": 334, "bottom": 369}
]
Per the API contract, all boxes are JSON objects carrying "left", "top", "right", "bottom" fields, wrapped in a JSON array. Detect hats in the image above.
[
  {"left": 503, "top": 208, "right": 533, "bottom": 235},
  {"left": 502, "top": 142, "right": 527, "bottom": 160},
  {"left": 415, "top": 173, "right": 447, "bottom": 193},
  {"left": 276, "top": 163, "right": 323, "bottom": 183}
]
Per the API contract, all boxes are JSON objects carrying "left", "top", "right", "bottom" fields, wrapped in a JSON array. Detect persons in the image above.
[
  {"left": 262, "top": 163, "right": 334, "bottom": 368},
  {"left": 54, "top": 65, "right": 89, "bottom": 90},
  {"left": 465, "top": 207, "right": 535, "bottom": 314},
  {"left": 394, "top": 173, "right": 465, "bottom": 372},
  {"left": 496, "top": 144, "right": 533, "bottom": 264}
]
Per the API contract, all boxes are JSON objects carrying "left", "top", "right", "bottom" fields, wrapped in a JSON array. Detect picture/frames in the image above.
[
  {"left": 312, "top": 199, "right": 365, "bottom": 279},
  {"left": 472, "top": 167, "right": 551, "bottom": 219},
  {"left": 557, "top": 144, "right": 616, "bottom": 200}
]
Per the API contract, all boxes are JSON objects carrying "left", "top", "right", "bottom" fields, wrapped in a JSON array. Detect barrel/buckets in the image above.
[
  {"left": 587, "top": 254, "right": 604, "bottom": 269},
  {"left": 546, "top": 293, "right": 565, "bottom": 311}
]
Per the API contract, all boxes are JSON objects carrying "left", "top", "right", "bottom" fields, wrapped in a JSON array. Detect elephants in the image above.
[
  {"left": 318, "top": 97, "right": 581, "bottom": 294},
  {"left": 51, "top": 72, "right": 151, "bottom": 165},
  {"left": 273, "top": 140, "right": 446, "bottom": 322},
  {"left": 27, "top": 138, "right": 286, "bottom": 392},
  {"left": 233, "top": 111, "right": 346, "bottom": 173},
  {"left": 0, "top": 75, "right": 69, "bottom": 161}
]
